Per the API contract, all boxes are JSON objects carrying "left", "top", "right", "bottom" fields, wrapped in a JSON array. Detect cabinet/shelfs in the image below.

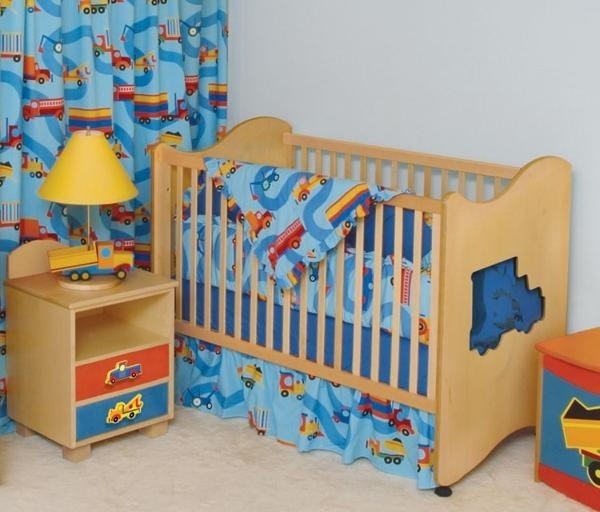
[
  {"left": 3, "top": 239, "right": 180, "bottom": 464},
  {"left": 535, "top": 326, "right": 600, "bottom": 511}
]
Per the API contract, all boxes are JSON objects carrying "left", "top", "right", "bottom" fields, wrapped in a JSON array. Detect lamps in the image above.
[{"left": 37, "top": 126, "right": 139, "bottom": 290}]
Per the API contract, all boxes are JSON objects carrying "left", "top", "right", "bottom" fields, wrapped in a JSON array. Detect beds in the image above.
[{"left": 150, "top": 116, "right": 572, "bottom": 497}]
